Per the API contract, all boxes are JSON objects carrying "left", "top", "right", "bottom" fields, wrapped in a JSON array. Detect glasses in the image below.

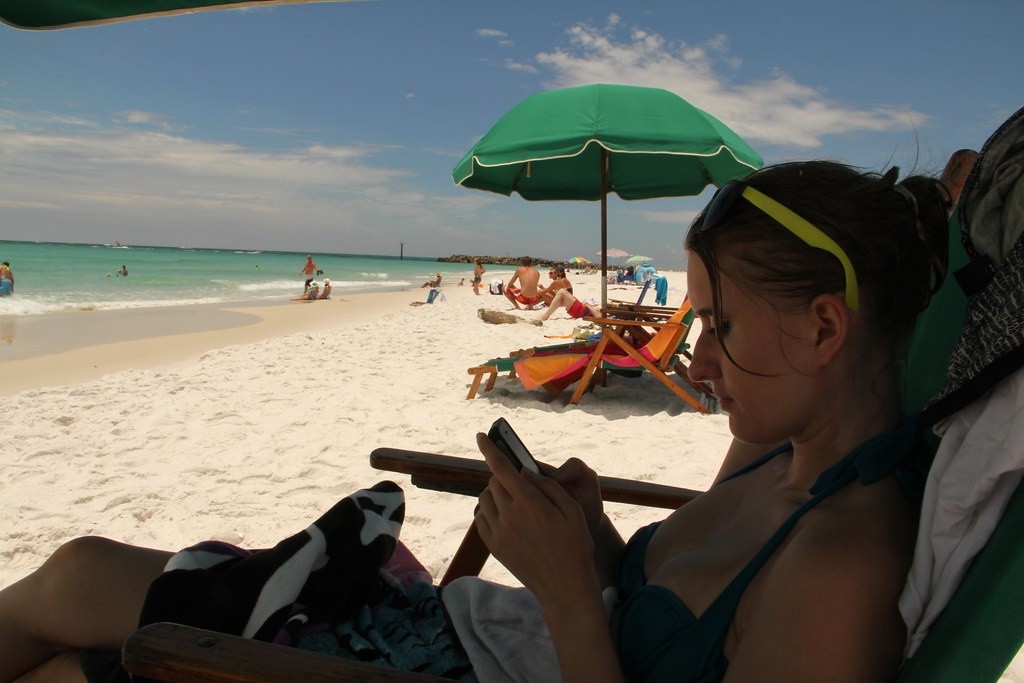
[{"left": 702, "top": 180, "right": 859, "bottom": 309}]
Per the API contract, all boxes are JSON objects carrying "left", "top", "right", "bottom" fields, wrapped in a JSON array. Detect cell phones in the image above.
[{"left": 488, "top": 418, "right": 545, "bottom": 478}]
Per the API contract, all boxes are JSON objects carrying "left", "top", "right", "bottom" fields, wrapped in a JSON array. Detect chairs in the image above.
[
  {"left": 467, "top": 294, "right": 717, "bottom": 414},
  {"left": 122, "top": 448, "right": 705, "bottom": 683}
]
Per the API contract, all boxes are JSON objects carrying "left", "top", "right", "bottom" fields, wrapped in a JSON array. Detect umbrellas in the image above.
[
  {"left": 625, "top": 256, "right": 652, "bottom": 264},
  {"left": 596, "top": 247, "right": 630, "bottom": 271},
  {"left": 564, "top": 256, "right": 589, "bottom": 271},
  {"left": 452, "top": 83, "right": 765, "bottom": 387},
  {"left": 635, "top": 264, "right": 657, "bottom": 285}
]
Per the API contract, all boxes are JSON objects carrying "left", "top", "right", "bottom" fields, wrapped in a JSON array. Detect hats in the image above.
[
  {"left": 324, "top": 279, "right": 331, "bottom": 284},
  {"left": 309, "top": 281, "right": 318, "bottom": 287}
]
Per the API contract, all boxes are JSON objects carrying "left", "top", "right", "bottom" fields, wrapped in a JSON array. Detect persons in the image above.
[
  {"left": 421, "top": 273, "right": 441, "bottom": 288},
  {"left": 0, "top": 262, "right": 14, "bottom": 296},
  {"left": 122, "top": 266, "right": 128, "bottom": 276},
  {"left": 316, "top": 279, "right": 332, "bottom": 298},
  {"left": 503, "top": 256, "right": 544, "bottom": 310},
  {"left": 293, "top": 282, "right": 319, "bottom": 300},
  {"left": 299, "top": 255, "right": 320, "bottom": 295},
  {"left": 473, "top": 258, "right": 485, "bottom": 295},
  {"left": 530, "top": 289, "right": 614, "bottom": 321},
  {"left": 628, "top": 267, "right": 634, "bottom": 275},
  {"left": 0, "top": 160, "right": 950, "bottom": 683},
  {"left": 534, "top": 266, "right": 572, "bottom": 306}
]
